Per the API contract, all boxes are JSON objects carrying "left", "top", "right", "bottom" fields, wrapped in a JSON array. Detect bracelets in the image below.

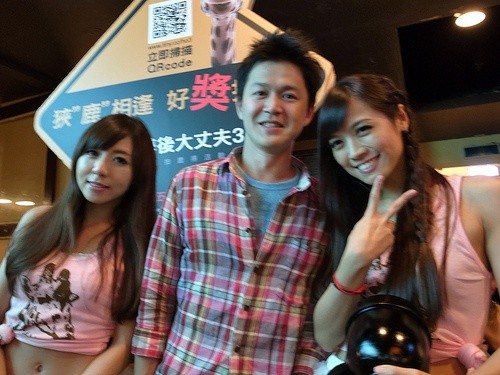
[{"left": 332, "top": 272, "right": 369, "bottom": 295}]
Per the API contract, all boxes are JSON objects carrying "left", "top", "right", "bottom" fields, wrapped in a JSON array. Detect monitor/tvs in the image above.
[{"left": 397, "top": 4, "right": 500, "bottom": 111}]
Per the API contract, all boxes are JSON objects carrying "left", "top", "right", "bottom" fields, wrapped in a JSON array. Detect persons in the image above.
[
  {"left": 317, "top": 76, "right": 500, "bottom": 375},
  {"left": 130, "top": 28, "right": 338, "bottom": 375},
  {"left": 0, "top": 114, "right": 157, "bottom": 375}
]
